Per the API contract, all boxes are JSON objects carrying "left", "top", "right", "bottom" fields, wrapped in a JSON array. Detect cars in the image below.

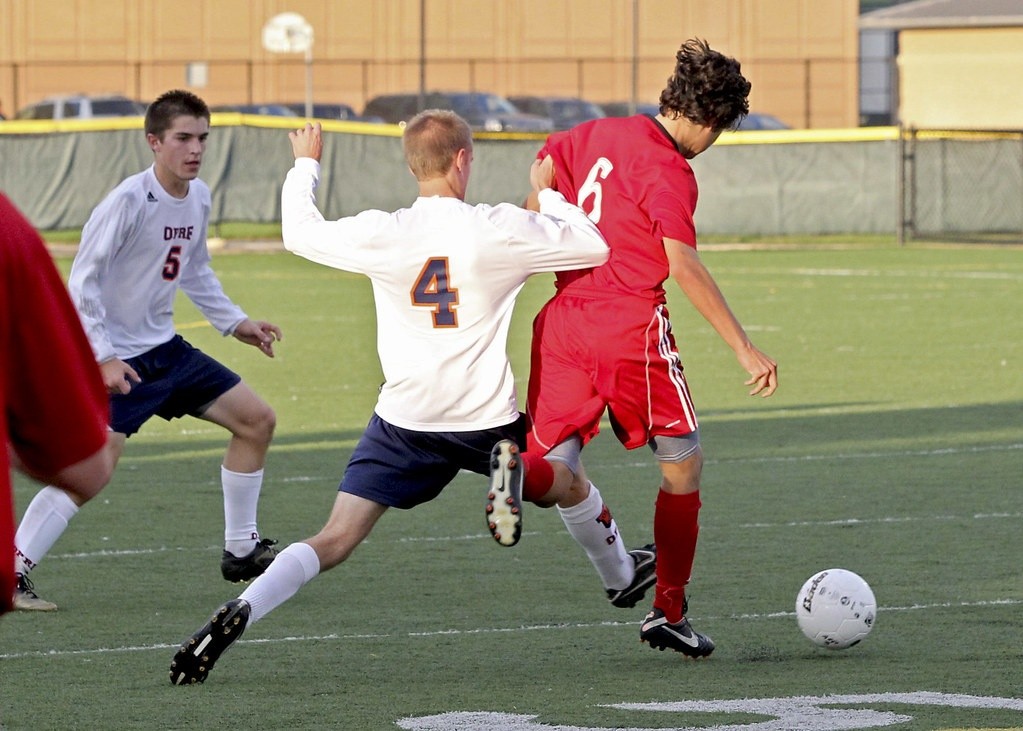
[
  {"left": 13, "top": 95, "right": 150, "bottom": 122},
  {"left": 494, "top": 93, "right": 788, "bottom": 132},
  {"left": 209, "top": 101, "right": 386, "bottom": 125},
  {"left": 360, "top": 90, "right": 555, "bottom": 136}
]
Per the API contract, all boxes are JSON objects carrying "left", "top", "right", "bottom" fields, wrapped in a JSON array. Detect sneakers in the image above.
[
  {"left": 221, "top": 537, "right": 281, "bottom": 585},
  {"left": 605, "top": 543, "right": 656, "bottom": 608},
  {"left": 640, "top": 593, "right": 715, "bottom": 659},
  {"left": 169, "top": 599, "right": 251, "bottom": 685},
  {"left": 11, "top": 572, "right": 58, "bottom": 612},
  {"left": 486, "top": 439, "right": 524, "bottom": 547}
]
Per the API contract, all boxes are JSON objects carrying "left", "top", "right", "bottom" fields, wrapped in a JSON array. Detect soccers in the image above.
[{"left": 794, "top": 567, "right": 878, "bottom": 650}]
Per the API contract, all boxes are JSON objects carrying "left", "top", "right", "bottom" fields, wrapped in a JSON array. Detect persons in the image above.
[
  {"left": 167, "top": 108, "right": 656, "bottom": 686},
  {"left": 0, "top": 91, "right": 283, "bottom": 619},
  {"left": 486, "top": 37, "right": 780, "bottom": 662}
]
